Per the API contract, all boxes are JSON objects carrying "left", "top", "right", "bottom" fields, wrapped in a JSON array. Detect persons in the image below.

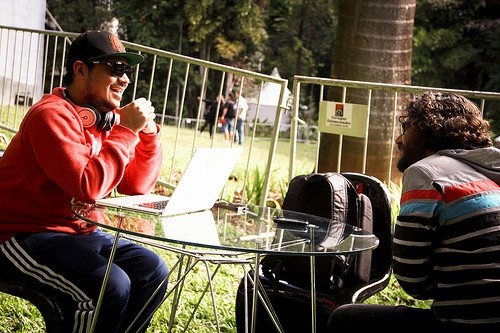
[
  {"left": 195, "top": 90, "right": 248, "bottom": 145},
  {"left": 329, "top": 91, "right": 500, "bottom": 333},
  {"left": 0, "top": 30, "right": 171, "bottom": 333}
]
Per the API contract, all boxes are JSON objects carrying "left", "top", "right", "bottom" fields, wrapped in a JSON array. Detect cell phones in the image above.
[
  {"left": 276, "top": 225, "right": 308, "bottom": 234},
  {"left": 273, "top": 216, "right": 309, "bottom": 225}
]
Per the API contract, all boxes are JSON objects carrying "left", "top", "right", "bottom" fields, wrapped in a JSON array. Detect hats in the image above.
[{"left": 67, "top": 30, "right": 144, "bottom": 65}]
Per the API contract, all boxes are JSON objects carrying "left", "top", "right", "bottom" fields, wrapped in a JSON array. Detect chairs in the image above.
[{"left": 235, "top": 172, "right": 395, "bottom": 333}]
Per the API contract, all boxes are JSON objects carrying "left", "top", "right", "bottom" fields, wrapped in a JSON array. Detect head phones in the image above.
[{"left": 61, "top": 88, "right": 116, "bottom": 131}]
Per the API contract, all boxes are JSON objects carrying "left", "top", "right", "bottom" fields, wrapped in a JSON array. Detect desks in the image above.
[{"left": 71, "top": 197, "right": 378, "bottom": 333}]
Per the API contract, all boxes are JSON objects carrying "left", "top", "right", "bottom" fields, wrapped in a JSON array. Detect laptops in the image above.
[{"left": 94, "top": 147, "right": 243, "bottom": 216}]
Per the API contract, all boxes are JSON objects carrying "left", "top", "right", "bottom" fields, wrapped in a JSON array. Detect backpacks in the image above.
[{"left": 261, "top": 172, "right": 372, "bottom": 290}]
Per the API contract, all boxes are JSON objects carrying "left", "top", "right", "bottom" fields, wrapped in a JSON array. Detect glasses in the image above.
[
  {"left": 88, "top": 61, "right": 134, "bottom": 77},
  {"left": 400, "top": 121, "right": 414, "bottom": 135}
]
